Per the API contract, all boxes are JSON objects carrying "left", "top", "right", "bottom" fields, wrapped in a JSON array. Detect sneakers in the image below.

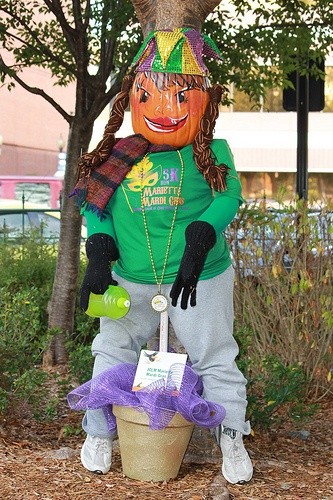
[
  {"left": 214, "top": 424, "right": 253, "bottom": 483},
  {"left": 80, "top": 434, "right": 112, "bottom": 474}
]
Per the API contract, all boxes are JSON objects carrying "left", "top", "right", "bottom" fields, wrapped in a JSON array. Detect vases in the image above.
[{"left": 112, "top": 404, "right": 196, "bottom": 482}]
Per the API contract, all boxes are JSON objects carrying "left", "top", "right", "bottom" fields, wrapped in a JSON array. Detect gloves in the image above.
[
  {"left": 168, "top": 220, "right": 216, "bottom": 312},
  {"left": 80, "top": 233, "right": 119, "bottom": 311}
]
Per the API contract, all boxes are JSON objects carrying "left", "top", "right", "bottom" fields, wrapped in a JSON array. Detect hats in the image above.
[{"left": 130, "top": 29, "right": 224, "bottom": 76}]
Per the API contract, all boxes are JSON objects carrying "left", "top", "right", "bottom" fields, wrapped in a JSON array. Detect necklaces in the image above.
[{"left": 139, "top": 149, "right": 185, "bottom": 312}]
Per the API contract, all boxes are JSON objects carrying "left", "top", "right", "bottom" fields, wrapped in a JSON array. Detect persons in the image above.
[{"left": 79, "top": 31, "right": 254, "bottom": 485}]
